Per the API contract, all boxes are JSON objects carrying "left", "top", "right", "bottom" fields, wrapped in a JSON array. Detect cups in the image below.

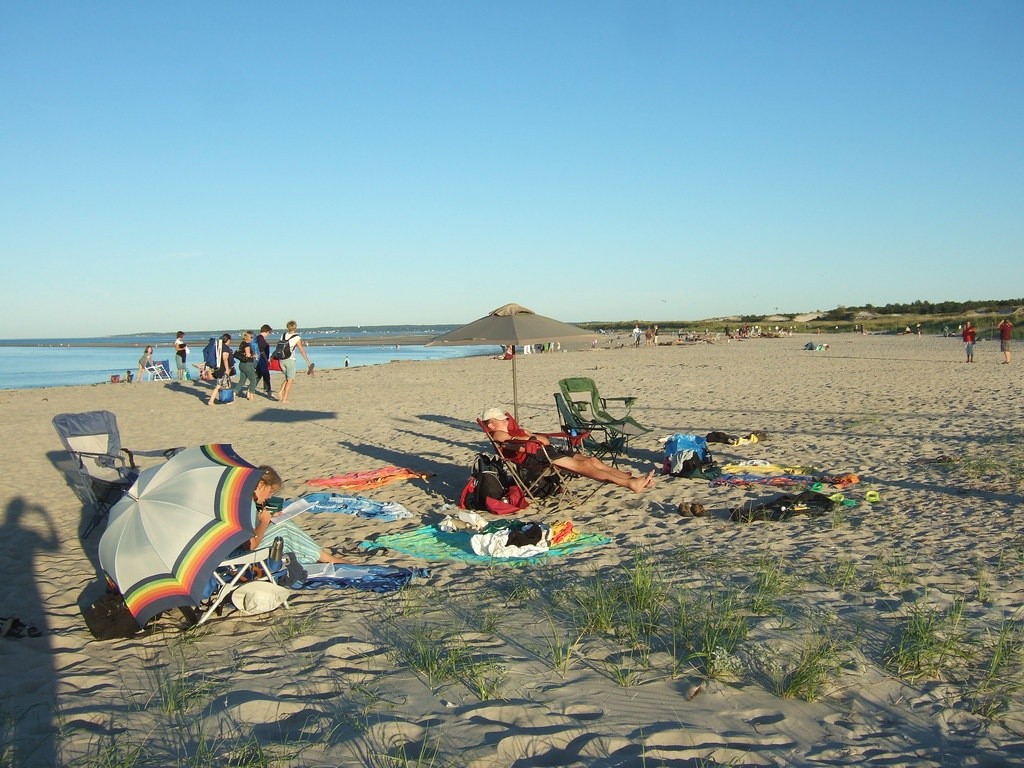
[{"left": 267, "top": 559, "right": 282, "bottom": 572}]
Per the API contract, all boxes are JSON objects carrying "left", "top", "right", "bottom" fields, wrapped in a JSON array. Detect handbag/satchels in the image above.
[
  {"left": 219, "top": 374, "right": 234, "bottom": 402},
  {"left": 233, "top": 350, "right": 248, "bottom": 363},
  {"left": 268, "top": 356, "right": 282, "bottom": 371}
]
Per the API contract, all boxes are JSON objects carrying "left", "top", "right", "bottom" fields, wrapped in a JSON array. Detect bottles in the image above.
[
  {"left": 706, "top": 453, "right": 712, "bottom": 461},
  {"left": 502, "top": 496, "right": 508, "bottom": 502},
  {"left": 255, "top": 350, "right": 258, "bottom": 356},
  {"left": 571, "top": 428, "right": 577, "bottom": 436},
  {"left": 521, "top": 524, "right": 532, "bottom": 531},
  {"left": 270, "top": 537, "right": 284, "bottom": 561},
  {"left": 539, "top": 524, "right": 549, "bottom": 530}
]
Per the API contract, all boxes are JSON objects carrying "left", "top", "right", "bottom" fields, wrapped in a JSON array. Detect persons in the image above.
[
  {"left": 855, "top": 323, "right": 867, "bottom": 333},
  {"left": 175, "top": 331, "right": 190, "bottom": 380},
  {"left": 343, "top": 356, "right": 350, "bottom": 368},
  {"left": 633, "top": 324, "right": 641, "bottom": 347},
  {"left": 229, "top": 466, "right": 352, "bottom": 575},
  {"left": 962, "top": 322, "right": 976, "bottom": 362},
  {"left": 645, "top": 325, "right": 658, "bottom": 347},
  {"left": 544, "top": 342, "right": 560, "bottom": 353},
  {"left": 136, "top": 346, "right": 153, "bottom": 382},
  {"left": 506, "top": 346, "right": 512, "bottom": 355},
  {"left": 723, "top": 324, "right": 730, "bottom": 337},
  {"left": 905, "top": 322, "right": 923, "bottom": 339},
  {"left": 203, "top": 338, "right": 218, "bottom": 367},
  {"left": 247, "top": 325, "right": 275, "bottom": 392},
  {"left": 482, "top": 408, "right": 655, "bottom": 493},
  {"left": 208, "top": 334, "right": 237, "bottom": 405},
  {"left": 278, "top": 321, "right": 314, "bottom": 402},
  {"left": 122, "top": 371, "right": 133, "bottom": 383},
  {"left": 997, "top": 319, "right": 1013, "bottom": 365},
  {"left": 671, "top": 327, "right": 708, "bottom": 338},
  {"left": 803, "top": 342, "right": 831, "bottom": 351},
  {"left": 234, "top": 330, "right": 262, "bottom": 401},
  {"left": 739, "top": 324, "right": 797, "bottom": 337}
]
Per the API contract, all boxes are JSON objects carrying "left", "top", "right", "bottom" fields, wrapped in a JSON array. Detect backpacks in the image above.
[
  {"left": 471, "top": 453, "right": 509, "bottom": 510},
  {"left": 276, "top": 333, "right": 300, "bottom": 359},
  {"left": 84, "top": 587, "right": 161, "bottom": 641}
]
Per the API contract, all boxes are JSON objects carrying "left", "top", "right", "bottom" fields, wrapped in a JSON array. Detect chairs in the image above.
[
  {"left": 143, "top": 360, "right": 172, "bottom": 382},
  {"left": 477, "top": 379, "right": 654, "bottom": 513},
  {"left": 52, "top": 411, "right": 187, "bottom": 540},
  {"left": 107, "top": 374, "right": 134, "bottom": 384},
  {"left": 195, "top": 548, "right": 289, "bottom": 625}
]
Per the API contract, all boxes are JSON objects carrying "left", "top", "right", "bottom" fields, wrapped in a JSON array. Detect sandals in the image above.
[{"left": 0, "top": 614, "right": 42, "bottom": 638}]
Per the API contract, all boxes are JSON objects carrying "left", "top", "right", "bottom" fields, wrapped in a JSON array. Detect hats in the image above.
[
  {"left": 231, "top": 581, "right": 289, "bottom": 615},
  {"left": 482, "top": 408, "right": 510, "bottom": 421}
]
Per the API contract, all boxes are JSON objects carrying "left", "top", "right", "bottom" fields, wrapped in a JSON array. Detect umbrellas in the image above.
[
  {"left": 98, "top": 445, "right": 267, "bottom": 629},
  {"left": 423, "top": 303, "right": 609, "bottom": 423}
]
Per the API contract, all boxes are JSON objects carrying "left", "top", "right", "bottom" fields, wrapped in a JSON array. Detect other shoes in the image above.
[
  {"left": 678, "top": 502, "right": 710, "bottom": 517},
  {"left": 307, "top": 363, "right": 314, "bottom": 375}
]
